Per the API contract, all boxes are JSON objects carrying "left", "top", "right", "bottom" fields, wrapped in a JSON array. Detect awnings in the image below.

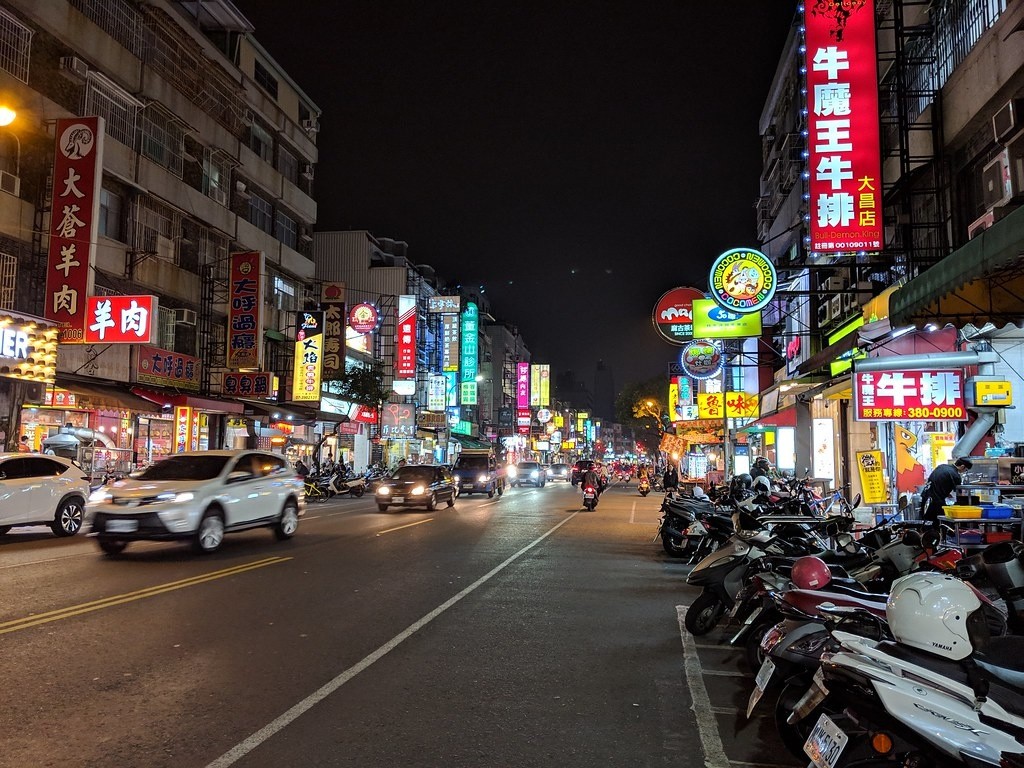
[
  {"left": 55, "top": 379, "right": 350, "bottom": 427},
  {"left": 796, "top": 206, "right": 1024, "bottom": 376}
]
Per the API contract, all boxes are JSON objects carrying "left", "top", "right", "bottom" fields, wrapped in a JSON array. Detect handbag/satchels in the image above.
[{"left": 665, "top": 489, "right": 676, "bottom": 499}]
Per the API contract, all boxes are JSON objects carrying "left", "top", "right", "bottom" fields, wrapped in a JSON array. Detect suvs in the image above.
[
  {"left": 88, "top": 450, "right": 305, "bottom": 555},
  {"left": 0, "top": 453, "right": 91, "bottom": 536},
  {"left": 572, "top": 460, "right": 595, "bottom": 485}
]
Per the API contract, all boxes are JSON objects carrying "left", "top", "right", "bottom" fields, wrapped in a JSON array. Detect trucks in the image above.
[{"left": 451, "top": 454, "right": 505, "bottom": 498}]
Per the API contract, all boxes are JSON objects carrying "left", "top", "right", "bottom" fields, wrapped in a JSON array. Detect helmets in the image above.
[
  {"left": 759, "top": 459, "right": 770, "bottom": 471},
  {"left": 588, "top": 463, "right": 597, "bottom": 468},
  {"left": 884, "top": 571, "right": 982, "bottom": 662},
  {"left": 754, "top": 476, "right": 770, "bottom": 491},
  {"left": 737, "top": 473, "right": 752, "bottom": 488},
  {"left": 692, "top": 486, "right": 703, "bottom": 497},
  {"left": 792, "top": 555, "right": 833, "bottom": 591}
]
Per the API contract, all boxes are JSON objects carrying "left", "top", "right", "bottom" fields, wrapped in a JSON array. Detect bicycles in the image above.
[{"left": 304, "top": 478, "right": 330, "bottom": 503}]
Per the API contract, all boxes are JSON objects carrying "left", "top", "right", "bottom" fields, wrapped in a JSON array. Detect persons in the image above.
[
  {"left": 296, "top": 452, "right": 335, "bottom": 478},
  {"left": 918, "top": 457, "right": 972, "bottom": 517},
  {"left": 582, "top": 450, "right": 681, "bottom": 513},
  {"left": 18, "top": 436, "right": 35, "bottom": 453}
]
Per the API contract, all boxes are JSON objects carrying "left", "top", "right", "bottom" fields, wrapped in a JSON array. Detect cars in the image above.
[
  {"left": 516, "top": 462, "right": 545, "bottom": 488},
  {"left": 375, "top": 465, "right": 457, "bottom": 511},
  {"left": 546, "top": 464, "right": 571, "bottom": 482}
]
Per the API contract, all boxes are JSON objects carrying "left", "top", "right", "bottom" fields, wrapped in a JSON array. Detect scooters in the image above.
[
  {"left": 653, "top": 456, "right": 1024, "bottom": 768},
  {"left": 583, "top": 467, "right": 664, "bottom": 511},
  {"left": 320, "top": 463, "right": 394, "bottom": 497}
]
